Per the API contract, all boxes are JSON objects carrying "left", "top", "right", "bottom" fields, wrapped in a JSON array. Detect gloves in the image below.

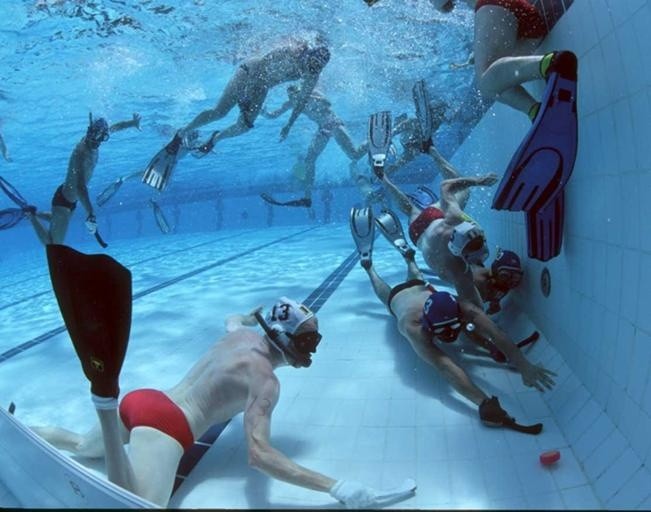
[
  {"left": 479, "top": 396, "right": 510, "bottom": 427},
  {"left": 238, "top": 92, "right": 251, "bottom": 109},
  {"left": 85, "top": 216, "right": 96, "bottom": 235},
  {"left": 485, "top": 339, "right": 508, "bottom": 363},
  {"left": 329, "top": 478, "right": 375, "bottom": 510}
]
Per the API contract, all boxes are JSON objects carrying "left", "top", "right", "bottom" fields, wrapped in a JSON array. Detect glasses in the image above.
[
  {"left": 466, "top": 237, "right": 484, "bottom": 253},
  {"left": 426, "top": 315, "right": 463, "bottom": 336},
  {"left": 291, "top": 332, "right": 321, "bottom": 354},
  {"left": 91, "top": 129, "right": 110, "bottom": 142},
  {"left": 497, "top": 268, "right": 521, "bottom": 285},
  {"left": 307, "top": 54, "right": 325, "bottom": 72}
]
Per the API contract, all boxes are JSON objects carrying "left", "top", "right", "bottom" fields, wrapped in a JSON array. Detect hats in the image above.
[
  {"left": 420, "top": 291, "right": 460, "bottom": 332},
  {"left": 448, "top": 221, "right": 489, "bottom": 266},
  {"left": 89, "top": 118, "right": 109, "bottom": 137},
  {"left": 491, "top": 250, "right": 521, "bottom": 286},
  {"left": 264, "top": 296, "right": 313, "bottom": 368},
  {"left": 298, "top": 43, "right": 330, "bottom": 79}
]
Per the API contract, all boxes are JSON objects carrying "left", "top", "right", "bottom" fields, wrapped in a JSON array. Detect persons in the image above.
[
  {"left": 259, "top": 85, "right": 370, "bottom": 204},
  {"left": 7, "top": 243, "right": 377, "bottom": 509},
  {"left": 176, "top": 37, "right": 330, "bottom": 154},
  {"left": 368, "top": 111, "right": 524, "bottom": 304},
  {"left": 382, "top": 98, "right": 453, "bottom": 184},
  {"left": 350, "top": 206, "right": 558, "bottom": 427},
  {"left": 24, "top": 112, "right": 142, "bottom": 245},
  {"left": 431, "top": 0, "right": 579, "bottom": 262}
]
